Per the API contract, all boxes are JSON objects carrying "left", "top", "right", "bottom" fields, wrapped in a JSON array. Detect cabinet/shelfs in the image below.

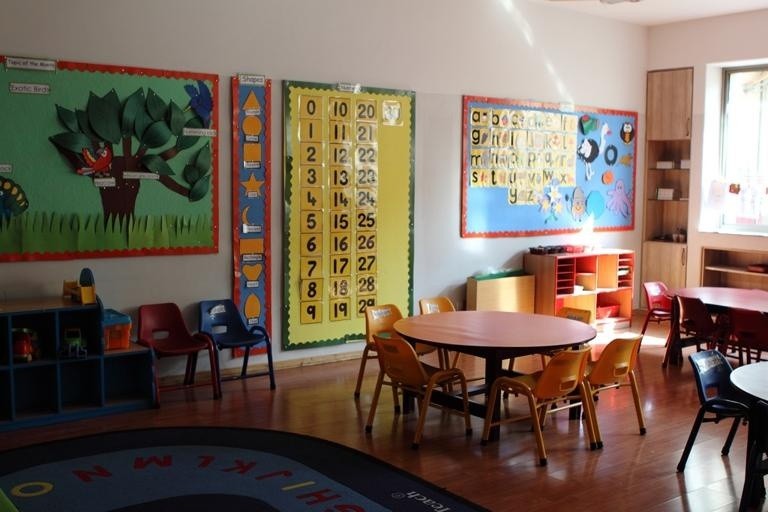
[
  {"left": 640, "top": 66, "right": 694, "bottom": 308},
  {"left": 524, "top": 247, "right": 634, "bottom": 333},
  {"left": 1, "top": 290, "right": 158, "bottom": 433},
  {"left": 699, "top": 244, "right": 767, "bottom": 287}
]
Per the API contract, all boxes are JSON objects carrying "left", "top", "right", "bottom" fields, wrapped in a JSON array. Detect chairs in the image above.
[
  {"left": 190, "top": 298, "right": 277, "bottom": 398},
  {"left": 420, "top": 295, "right": 486, "bottom": 383},
  {"left": 641, "top": 280, "right": 688, "bottom": 346},
  {"left": 354, "top": 305, "right": 437, "bottom": 413},
  {"left": 136, "top": 302, "right": 220, "bottom": 410},
  {"left": 582, "top": 334, "right": 645, "bottom": 446},
  {"left": 662, "top": 295, "right": 715, "bottom": 369},
  {"left": 729, "top": 307, "right": 768, "bottom": 365},
  {"left": 676, "top": 349, "right": 768, "bottom": 472},
  {"left": 508, "top": 307, "right": 591, "bottom": 371},
  {"left": 366, "top": 334, "right": 473, "bottom": 448},
  {"left": 480, "top": 346, "right": 599, "bottom": 467}
]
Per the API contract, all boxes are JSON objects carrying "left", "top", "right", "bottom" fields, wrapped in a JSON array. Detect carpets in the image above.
[{"left": 0, "top": 425, "right": 491, "bottom": 512}]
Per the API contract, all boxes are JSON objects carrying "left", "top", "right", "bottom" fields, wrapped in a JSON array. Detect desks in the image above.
[
  {"left": 728, "top": 360, "right": 768, "bottom": 512},
  {"left": 394, "top": 310, "right": 597, "bottom": 440},
  {"left": 669, "top": 287, "right": 768, "bottom": 367}
]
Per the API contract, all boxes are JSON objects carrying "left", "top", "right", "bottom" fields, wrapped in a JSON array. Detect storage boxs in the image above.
[{"left": 103, "top": 308, "right": 132, "bottom": 351}]
